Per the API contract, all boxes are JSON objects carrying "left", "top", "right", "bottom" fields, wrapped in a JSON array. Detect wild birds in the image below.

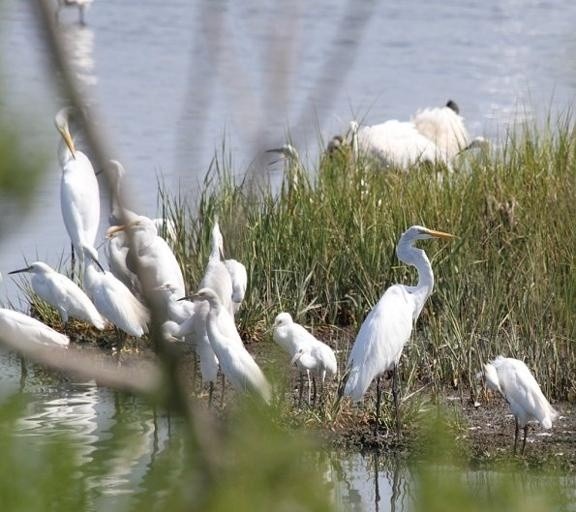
[
  {"left": 94, "top": 160, "right": 140, "bottom": 249},
  {"left": 0, "top": 215, "right": 274, "bottom": 410},
  {"left": 264, "top": 99, "right": 493, "bottom": 214},
  {"left": 53, "top": 103, "right": 107, "bottom": 281},
  {"left": 265, "top": 308, "right": 340, "bottom": 413},
  {"left": 332, "top": 222, "right": 463, "bottom": 445},
  {"left": 482, "top": 352, "right": 559, "bottom": 458}
]
